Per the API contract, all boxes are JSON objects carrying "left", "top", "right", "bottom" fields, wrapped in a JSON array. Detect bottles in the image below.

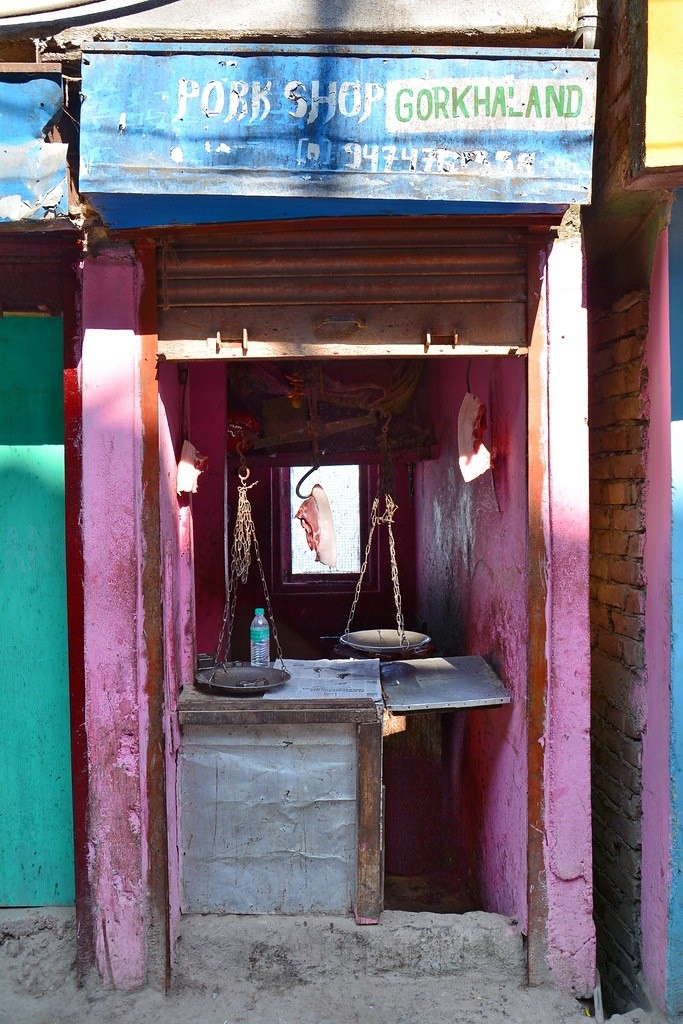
[{"left": 250, "top": 608, "right": 270, "bottom": 668}]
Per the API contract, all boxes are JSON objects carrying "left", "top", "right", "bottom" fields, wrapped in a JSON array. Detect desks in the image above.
[{"left": 178, "top": 656, "right": 383, "bottom": 924}]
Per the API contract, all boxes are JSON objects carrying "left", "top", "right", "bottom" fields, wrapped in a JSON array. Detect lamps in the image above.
[{"left": 285, "top": 360, "right": 307, "bottom": 408}]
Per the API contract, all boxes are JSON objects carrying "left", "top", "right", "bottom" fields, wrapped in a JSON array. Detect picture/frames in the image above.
[{"left": 271, "top": 464, "right": 388, "bottom": 599}]
